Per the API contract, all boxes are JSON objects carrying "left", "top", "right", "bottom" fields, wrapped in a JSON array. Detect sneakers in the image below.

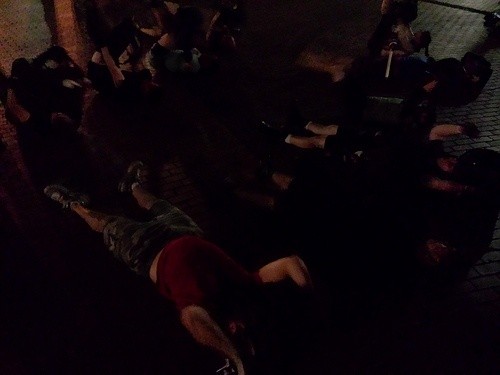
[
  {"left": 119, "top": 161, "right": 144, "bottom": 191},
  {"left": 44, "top": 184, "right": 89, "bottom": 210}
]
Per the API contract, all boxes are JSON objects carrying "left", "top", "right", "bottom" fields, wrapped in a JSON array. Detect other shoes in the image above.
[{"left": 255, "top": 119, "right": 285, "bottom": 144}]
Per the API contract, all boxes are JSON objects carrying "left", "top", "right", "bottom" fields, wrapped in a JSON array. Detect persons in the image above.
[
  {"left": 266, "top": 103, "right": 486, "bottom": 196},
  {"left": 40, "top": 159, "right": 311, "bottom": 375},
  {"left": 0, "top": 0, "right": 248, "bottom": 139},
  {"left": 328, "top": 0, "right": 488, "bottom": 123}
]
